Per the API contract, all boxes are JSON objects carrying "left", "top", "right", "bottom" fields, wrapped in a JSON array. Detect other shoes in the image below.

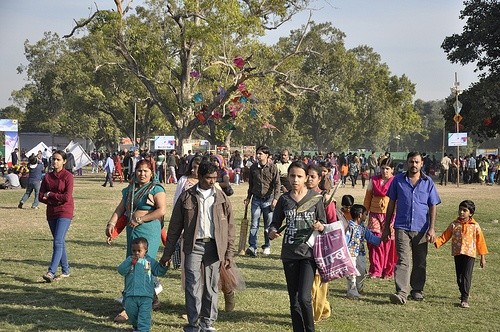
[
  {"left": 410, "top": 289, "right": 424, "bottom": 301},
  {"left": 461, "top": 302, "right": 469, "bottom": 308},
  {"left": 390, "top": 294, "right": 405, "bottom": 305},
  {"left": 114, "top": 298, "right": 123, "bottom": 303},
  {"left": 59, "top": 274, "right": 70, "bottom": 278},
  {"left": 43, "top": 272, "right": 54, "bottom": 282},
  {"left": 152, "top": 301, "right": 161, "bottom": 310},
  {"left": 18, "top": 200, "right": 23, "bottom": 208},
  {"left": 31, "top": 206, "right": 40, "bottom": 209},
  {"left": 246, "top": 246, "right": 258, "bottom": 257},
  {"left": 263, "top": 247, "right": 270, "bottom": 254},
  {"left": 114, "top": 310, "right": 129, "bottom": 321}
]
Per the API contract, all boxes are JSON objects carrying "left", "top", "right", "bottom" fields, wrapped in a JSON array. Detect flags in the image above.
[{"left": 25, "top": 140, "right": 93, "bottom": 172}]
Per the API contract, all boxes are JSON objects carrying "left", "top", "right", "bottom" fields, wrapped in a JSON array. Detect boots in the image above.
[{"left": 221, "top": 289, "right": 235, "bottom": 312}]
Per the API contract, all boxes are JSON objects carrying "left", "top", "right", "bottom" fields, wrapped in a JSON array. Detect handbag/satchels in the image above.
[
  {"left": 306, "top": 220, "right": 361, "bottom": 283},
  {"left": 145, "top": 193, "right": 155, "bottom": 207}
]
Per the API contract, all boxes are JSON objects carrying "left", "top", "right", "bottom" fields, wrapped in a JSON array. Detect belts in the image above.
[{"left": 196, "top": 237, "right": 215, "bottom": 243}]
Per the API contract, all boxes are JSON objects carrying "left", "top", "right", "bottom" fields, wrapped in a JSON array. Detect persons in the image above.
[
  {"left": 240, "top": 144, "right": 281, "bottom": 257},
  {"left": 166, "top": 149, "right": 257, "bottom": 185},
  {"left": 379, "top": 151, "right": 441, "bottom": 303},
  {"left": 422, "top": 152, "right": 500, "bottom": 187},
  {"left": 364, "top": 158, "right": 398, "bottom": 280},
  {"left": 159, "top": 161, "right": 236, "bottom": 332},
  {"left": 269, "top": 149, "right": 406, "bottom": 196},
  {"left": 18, "top": 157, "right": 44, "bottom": 209},
  {"left": 267, "top": 161, "right": 326, "bottom": 332},
  {"left": 104, "top": 159, "right": 167, "bottom": 321},
  {"left": 118, "top": 237, "right": 170, "bottom": 332},
  {"left": 78, "top": 147, "right": 167, "bottom": 187},
  {"left": 337, "top": 195, "right": 354, "bottom": 230},
  {"left": 305, "top": 164, "right": 338, "bottom": 323},
  {"left": 4, "top": 147, "right": 35, "bottom": 189},
  {"left": 180, "top": 155, "right": 244, "bottom": 321},
  {"left": 37, "top": 148, "right": 75, "bottom": 281},
  {"left": 430, "top": 199, "right": 490, "bottom": 308},
  {"left": 343, "top": 203, "right": 388, "bottom": 297}
]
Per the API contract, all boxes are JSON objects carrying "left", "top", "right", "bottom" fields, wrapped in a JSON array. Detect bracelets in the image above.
[
  {"left": 136, "top": 216, "right": 144, "bottom": 225},
  {"left": 107, "top": 221, "right": 117, "bottom": 227}
]
